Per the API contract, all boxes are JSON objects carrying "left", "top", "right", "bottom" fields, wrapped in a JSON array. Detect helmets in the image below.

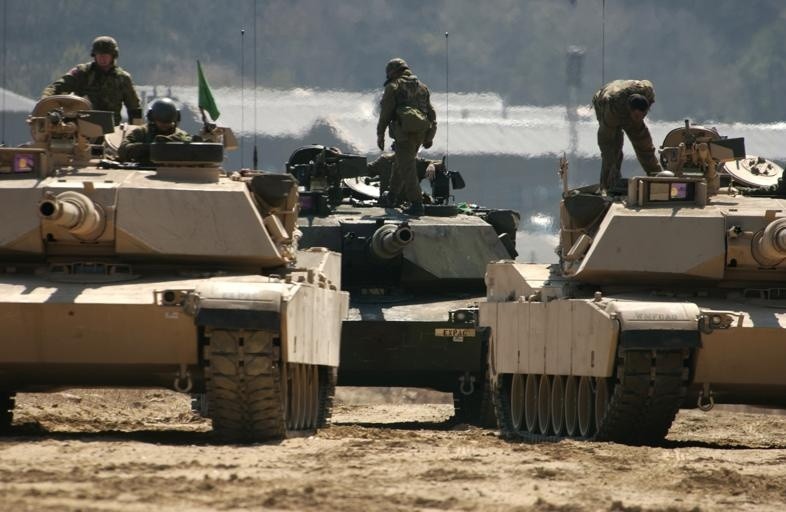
[
  {"left": 384, "top": 58, "right": 410, "bottom": 81},
  {"left": 145, "top": 97, "right": 182, "bottom": 126},
  {"left": 90, "top": 36, "right": 123, "bottom": 60}
]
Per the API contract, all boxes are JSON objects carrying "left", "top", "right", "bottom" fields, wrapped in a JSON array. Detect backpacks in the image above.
[{"left": 389, "top": 103, "right": 431, "bottom": 149}]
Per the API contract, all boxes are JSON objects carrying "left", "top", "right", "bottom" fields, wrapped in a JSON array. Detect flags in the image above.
[{"left": 197, "top": 60, "right": 220, "bottom": 121}]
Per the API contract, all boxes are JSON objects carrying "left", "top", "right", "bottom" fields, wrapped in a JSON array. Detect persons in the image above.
[
  {"left": 592, "top": 79, "right": 663, "bottom": 194},
  {"left": 41, "top": 36, "right": 142, "bottom": 126},
  {"left": 375, "top": 58, "right": 437, "bottom": 214},
  {"left": 330, "top": 141, "right": 443, "bottom": 197},
  {"left": 117, "top": 97, "right": 192, "bottom": 162}
]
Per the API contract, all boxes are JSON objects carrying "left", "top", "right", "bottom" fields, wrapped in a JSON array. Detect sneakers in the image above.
[
  {"left": 382, "top": 193, "right": 400, "bottom": 208},
  {"left": 402, "top": 201, "right": 425, "bottom": 216}
]
